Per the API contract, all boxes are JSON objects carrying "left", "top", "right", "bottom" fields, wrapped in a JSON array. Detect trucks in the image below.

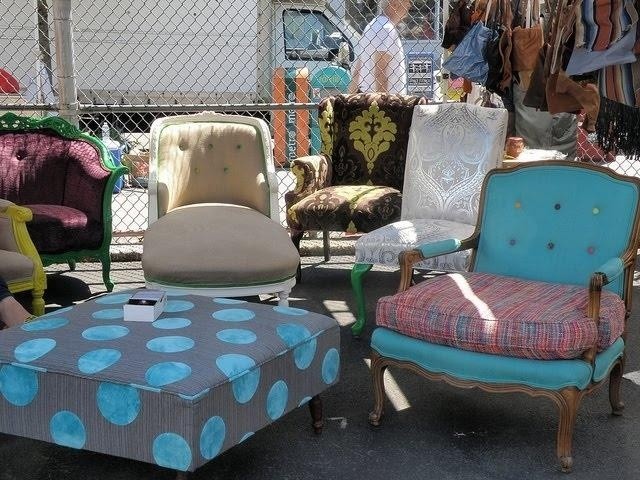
[{"left": 1, "top": 0, "right": 360, "bottom": 105}]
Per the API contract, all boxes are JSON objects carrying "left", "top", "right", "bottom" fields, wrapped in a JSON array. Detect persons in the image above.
[
  {"left": 1, "top": 274, "right": 32, "bottom": 327},
  {"left": 346, "top": 0, "right": 411, "bottom": 97}
]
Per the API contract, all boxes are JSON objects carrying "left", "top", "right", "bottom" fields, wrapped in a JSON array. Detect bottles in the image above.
[{"left": 102, "top": 122, "right": 110, "bottom": 146}]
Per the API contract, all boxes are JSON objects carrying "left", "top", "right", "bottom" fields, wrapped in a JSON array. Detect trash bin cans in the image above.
[
  {"left": 102, "top": 140, "right": 124, "bottom": 194},
  {"left": 273, "top": 67, "right": 353, "bottom": 166}
]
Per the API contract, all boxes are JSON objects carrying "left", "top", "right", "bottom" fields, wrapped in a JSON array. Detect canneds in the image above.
[{"left": 506, "top": 137, "right": 524, "bottom": 158}]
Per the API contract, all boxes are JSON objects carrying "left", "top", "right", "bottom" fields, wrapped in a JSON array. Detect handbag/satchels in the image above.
[{"left": 441, "top": 0, "right": 601, "bottom": 115}]
